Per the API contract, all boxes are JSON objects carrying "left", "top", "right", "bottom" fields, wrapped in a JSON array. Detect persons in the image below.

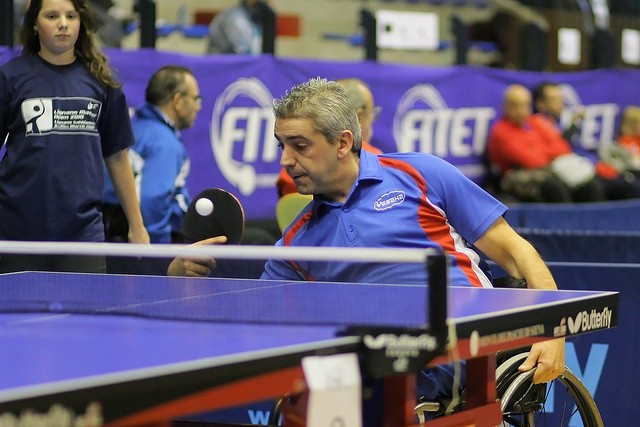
[
  {"left": 597, "top": 104, "right": 640, "bottom": 180},
  {"left": 166, "top": 77, "right": 566, "bottom": 423},
  {"left": 488, "top": 84, "right": 607, "bottom": 201},
  {"left": 276, "top": 78, "right": 385, "bottom": 198},
  {"left": 203, "top": 0, "right": 276, "bottom": 55},
  {"left": 102, "top": 66, "right": 203, "bottom": 243},
  {"left": 0, "top": 0, "right": 151, "bottom": 273},
  {"left": 532, "top": 83, "right": 631, "bottom": 183}
]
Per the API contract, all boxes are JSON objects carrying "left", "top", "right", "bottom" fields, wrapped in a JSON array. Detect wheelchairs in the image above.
[{"left": 267, "top": 277, "right": 603, "bottom": 427}]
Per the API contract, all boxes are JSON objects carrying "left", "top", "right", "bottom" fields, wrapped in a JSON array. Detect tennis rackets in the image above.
[{"left": 181, "top": 187, "right": 244, "bottom": 244}]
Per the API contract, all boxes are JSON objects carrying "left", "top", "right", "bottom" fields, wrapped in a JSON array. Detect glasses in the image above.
[{"left": 180, "top": 93, "right": 203, "bottom": 104}]
